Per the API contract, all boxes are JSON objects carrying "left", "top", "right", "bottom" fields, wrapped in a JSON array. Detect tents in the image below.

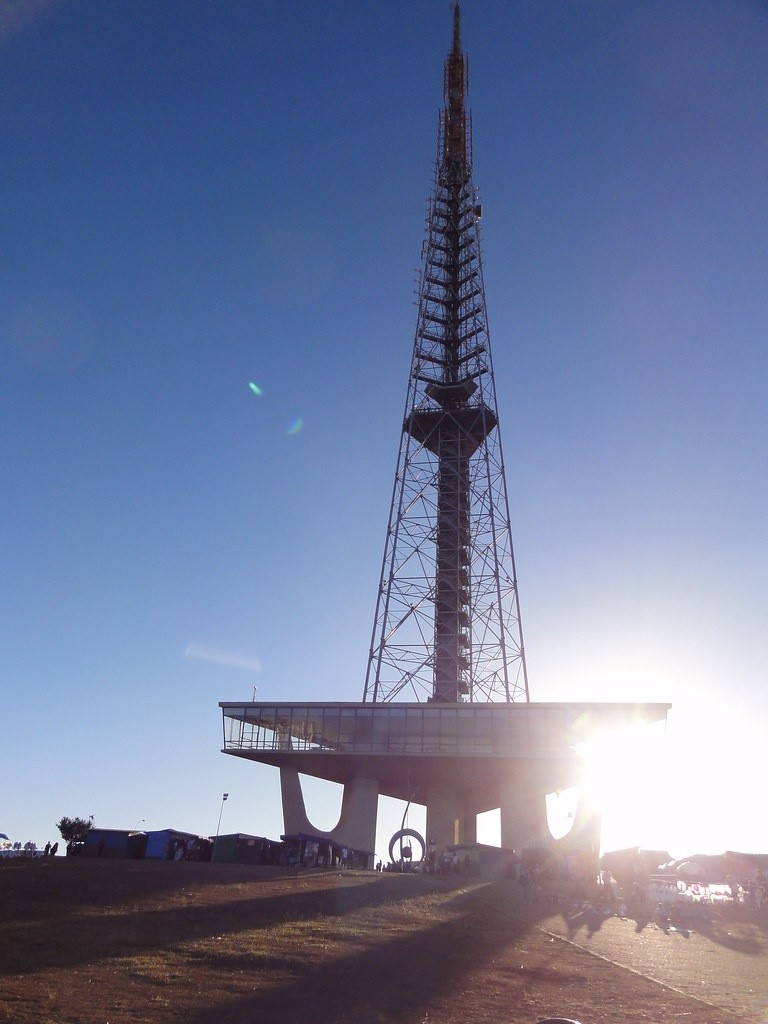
[{"left": 444, "top": 842, "right": 516, "bottom": 880}]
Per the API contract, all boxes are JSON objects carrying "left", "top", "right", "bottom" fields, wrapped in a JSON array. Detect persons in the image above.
[
  {"left": 45, "top": 841, "right": 51, "bottom": 855},
  {"left": 50, "top": 842, "right": 58, "bottom": 856},
  {"left": 428, "top": 849, "right": 470, "bottom": 878}
]
